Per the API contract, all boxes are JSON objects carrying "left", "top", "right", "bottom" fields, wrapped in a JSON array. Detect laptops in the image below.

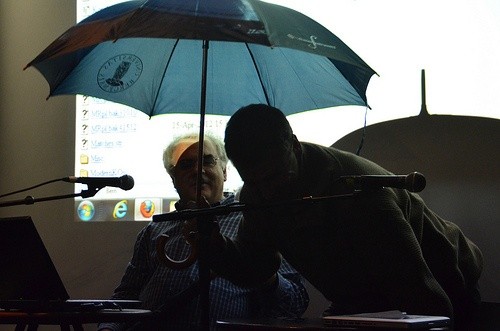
[
  {"left": 0, "top": 216, "right": 142, "bottom": 309},
  {"left": 320, "top": 314, "right": 450, "bottom": 329}
]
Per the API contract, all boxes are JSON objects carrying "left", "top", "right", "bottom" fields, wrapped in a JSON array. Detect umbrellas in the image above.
[{"left": 24, "top": 0, "right": 379, "bottom": 270}]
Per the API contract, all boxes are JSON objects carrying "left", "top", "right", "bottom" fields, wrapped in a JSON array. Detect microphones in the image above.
[
  {"left": 360, "top": 172, "right": 426, "bottom": 192},
  {"left": 64, "top": 175, "right": 134, "bottom": 190}
]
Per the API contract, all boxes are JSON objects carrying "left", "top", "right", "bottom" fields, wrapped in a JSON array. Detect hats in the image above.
[{"left": 162, "top": 133, "right": 199, "bottom": 170}]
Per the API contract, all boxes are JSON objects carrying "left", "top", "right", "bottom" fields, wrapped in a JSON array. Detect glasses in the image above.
[{"left": 179, "top": 156, "right": 221, "bottom": 172}]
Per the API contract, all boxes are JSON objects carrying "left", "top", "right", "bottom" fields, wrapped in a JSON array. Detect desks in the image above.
[
  {"left": 0, "top": 309, "right": 151, "bottom": 331},
  {"left": 216, "top": 320, "right": 357, "bottom": 331}
]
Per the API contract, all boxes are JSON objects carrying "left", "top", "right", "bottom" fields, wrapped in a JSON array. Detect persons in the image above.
[
  {"left": 98, "top": 133, "right": 309, "bottom": 330},
  {"left": 180, "top": 104, "right": 484, "bottom": 330}
]
